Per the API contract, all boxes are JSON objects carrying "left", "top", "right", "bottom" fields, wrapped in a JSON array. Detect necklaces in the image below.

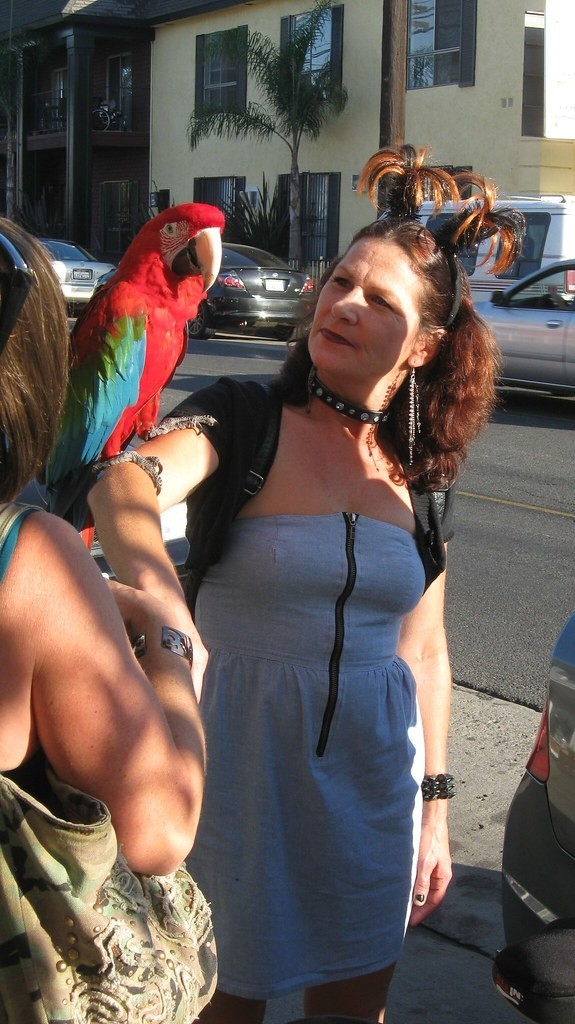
[{"left": 308, "top": 368, "right": 390, "bottom": 424}]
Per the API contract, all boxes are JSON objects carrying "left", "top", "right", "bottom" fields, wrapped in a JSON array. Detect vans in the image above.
[{"left": 378, "top": 193, "right": 574, "bottom": 303}]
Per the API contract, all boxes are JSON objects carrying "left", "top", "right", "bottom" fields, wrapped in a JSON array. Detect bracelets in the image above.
[
  {"left": 132, "top": 625, "right": 193, "bottom": 667},
  {"left": 422, "top": 774, "right": 455, "bottom": 800}
]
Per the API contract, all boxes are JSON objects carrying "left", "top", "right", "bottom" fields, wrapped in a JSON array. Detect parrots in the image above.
[{"left": 45, "top": 204, "right": 223, "bottom": 555}]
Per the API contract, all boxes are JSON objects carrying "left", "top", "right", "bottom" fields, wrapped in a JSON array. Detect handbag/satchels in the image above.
[{"left": 0, "top": 773, "right": 218, "bottom": 1024}]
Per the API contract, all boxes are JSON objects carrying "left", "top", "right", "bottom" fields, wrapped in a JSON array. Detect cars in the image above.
[
  {"left": 185, "top": 241, "right": 315, "bottom": 339},
  {"left": 471, "top": 259, "right": 574, "bottom": 393},
  {"left": 0, "top": 232, "right": 115, "bottom": 305}
]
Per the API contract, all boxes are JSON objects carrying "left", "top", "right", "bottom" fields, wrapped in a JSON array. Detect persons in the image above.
[
  {"left": 551, "top": 292, "right": 575, "bottom": 311},
  {"left": 0, "top": 216, "right": 206, "bottom": 1024},
  {"left": 86, "top": 145, "right": 524, "bottom": 1023}
]
